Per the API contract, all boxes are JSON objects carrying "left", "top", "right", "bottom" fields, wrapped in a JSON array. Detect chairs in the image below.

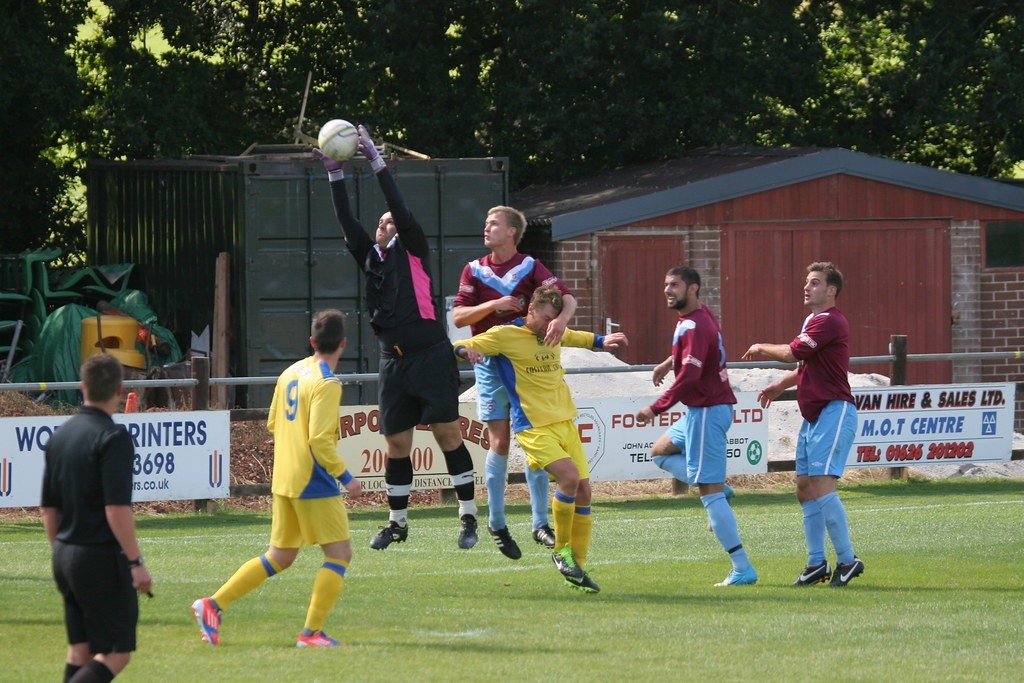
[{"left": 0, "top": 247, "right": 130, "bottom": 381}]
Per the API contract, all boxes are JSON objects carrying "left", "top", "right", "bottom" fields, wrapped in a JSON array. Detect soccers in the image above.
[{"left": 318, "top": 118, "right": 359, "bottom": 161}]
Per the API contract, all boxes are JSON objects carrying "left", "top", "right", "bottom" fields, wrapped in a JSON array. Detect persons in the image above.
[
  {"left": 40, "top": 354, "right": 152, "bottom": 683},
  {"left": 742, "top": 262, "right": 866, "bottom": 587},
  {"left": 191, "top": 310, "right": 362, "bottom": 647},
  {"left": 312, "top": 125, "right": 479, "bottom": 551},
  {"left": 452, "top": 286, "right": 628, "bottom": 593},
  {"left": 452, "top": 206, "right": 577, "bottom": 560},
  {"left": 636, "top": 267, "right": 757, "bottom": 586}
]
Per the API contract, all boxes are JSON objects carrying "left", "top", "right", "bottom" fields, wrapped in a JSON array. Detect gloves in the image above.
[
  {"left": 356, "top": 125, "right": 385, "bottom": 171},
  {"left": 312, "top": 148, "right": 347, "bottom": 182}
]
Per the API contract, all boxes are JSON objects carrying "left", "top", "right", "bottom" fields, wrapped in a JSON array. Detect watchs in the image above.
[{"left": 128, "top": 557, "right": 143, "bottom": 565}]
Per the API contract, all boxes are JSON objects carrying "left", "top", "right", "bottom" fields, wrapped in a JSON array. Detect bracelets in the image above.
[{"left": 131, "top": 565, "right": 138, "bottom": 568}]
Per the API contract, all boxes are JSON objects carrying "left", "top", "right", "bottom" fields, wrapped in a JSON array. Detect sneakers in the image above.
[
  {"left": 713, "top": 563, "right": 758, "bottom": 586},
  {"left": 296, "top": 628, "right": 345, "bottom": 649},
  {"left": 532, "top": 521, "right": 568, "bottom": 549},
  {"left": 191, "top": 592, "right": 223, "bottom": 648},
  {"left": 552, "top": 542, "right": 584, "bottom": 583},
  {"left": 563, "top": 569, "right": 600, "bottom": 594},
  {"left": 707, "top": 484, "right": 735, "bottom": 531},
  {"left": 369, "top": 520, "right": 408, "bottom": 551},
  {"left": 791, "top": 560, "right": 832, "bottom": 587},
  {"left": 487, "top": 521, "right": 521, "bottom": 559},
  {"left": 827, "top": 552, "right": 864, "bottom": 587},
  {"left": 458, "top": 514, "right": 478, "bottom": 549}
]
